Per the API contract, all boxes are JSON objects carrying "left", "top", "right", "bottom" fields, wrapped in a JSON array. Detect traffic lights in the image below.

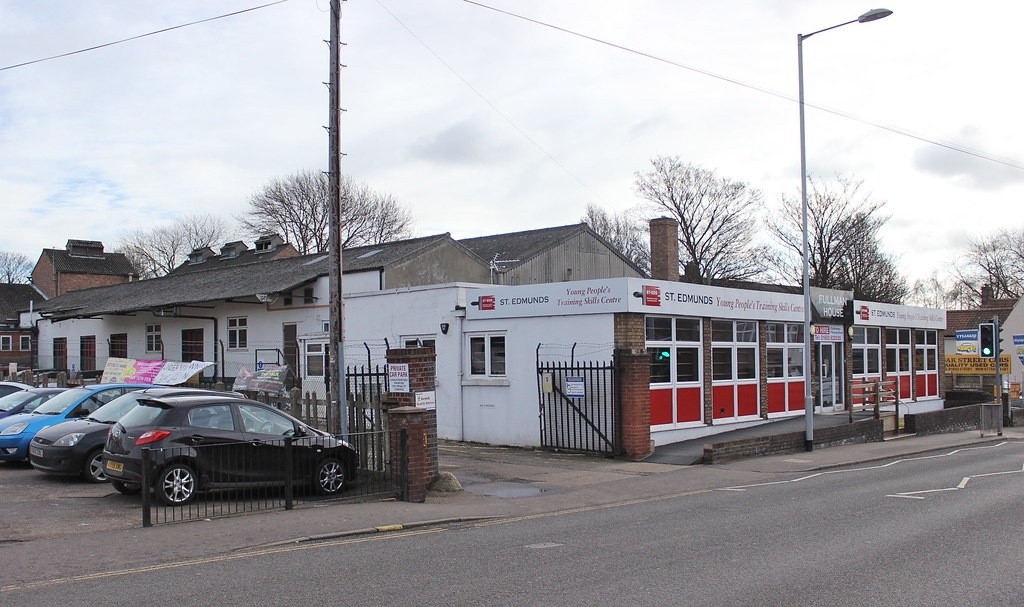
[{"left": 980, "top": 323, "right": 996, "bottom": 358}]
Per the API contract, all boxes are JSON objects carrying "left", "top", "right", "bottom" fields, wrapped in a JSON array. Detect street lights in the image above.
[{"left": 797, "top": 8, "right": 893, "bottom": 450}]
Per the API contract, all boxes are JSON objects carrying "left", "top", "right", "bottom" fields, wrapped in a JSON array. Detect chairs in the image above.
[
  {"left": 197, "top": 409, "right": 210, "bottom": 425},
  {"left": 101, "top": 394, "right": 110, "bottom": 403}
]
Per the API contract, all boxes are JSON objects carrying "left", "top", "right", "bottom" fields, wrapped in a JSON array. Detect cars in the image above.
[
  {"left": 0, "top": 382, "right": 165, "bottom": 469},
  {"left": 103, "top": 396, "right": 358, "bottom": 507},
  {"left": 30, "top": 388, "right": 247, "bottom": 482}
]
